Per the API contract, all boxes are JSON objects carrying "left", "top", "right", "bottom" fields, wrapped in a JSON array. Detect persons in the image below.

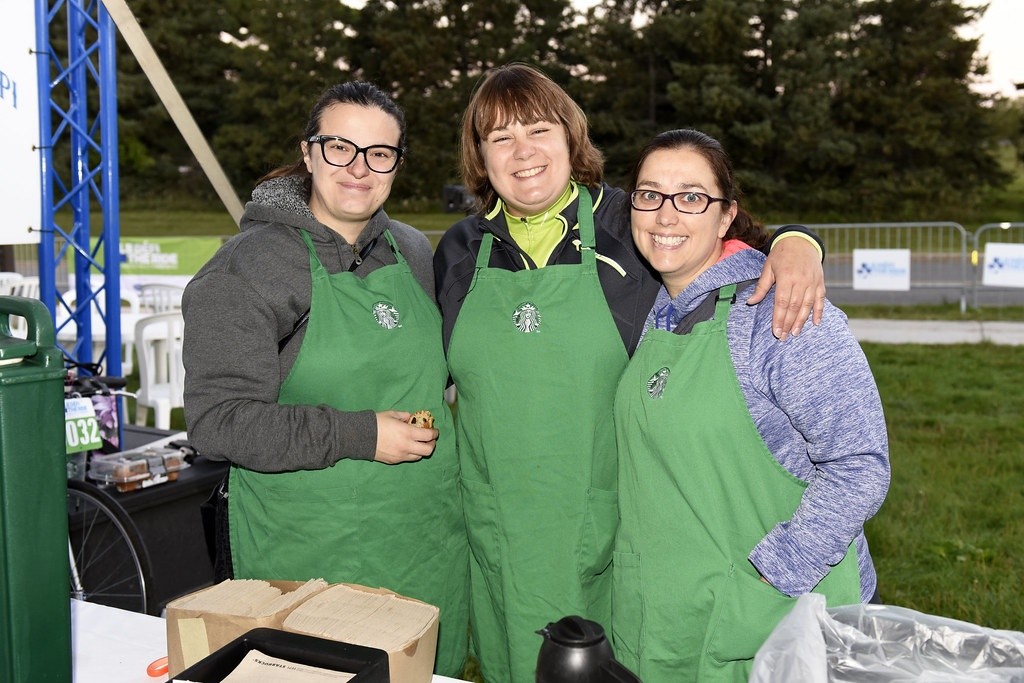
[
  {"left": 181, "top": 81, "right": 470, "bottom": 678},
  {"left": 613, "top": 129, "right": 892, "bottom": 682},
  {"left": 433, "top": 64, "right": 826, "bottom": 683}
]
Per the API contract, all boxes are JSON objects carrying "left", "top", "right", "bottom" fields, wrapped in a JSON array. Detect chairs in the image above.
[{"left": 0, "top": 271, "right": 184, "bottom": 430}]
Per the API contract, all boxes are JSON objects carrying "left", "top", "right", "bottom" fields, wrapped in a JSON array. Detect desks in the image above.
[{"left": 51, "top": 314, "right": 154, "bottom": 378}]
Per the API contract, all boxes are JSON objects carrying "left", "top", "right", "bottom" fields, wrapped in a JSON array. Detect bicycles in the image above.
[{"left": 60, "top": 375, "right": 159, "bottom": 619}]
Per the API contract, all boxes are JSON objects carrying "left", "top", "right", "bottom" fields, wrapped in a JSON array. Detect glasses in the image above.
[
  {"left": 630, "top": 190, "right": 730, "bottom": 214},
  {"left": 307, "top": 135, "right": 404, "bottom": 173}
]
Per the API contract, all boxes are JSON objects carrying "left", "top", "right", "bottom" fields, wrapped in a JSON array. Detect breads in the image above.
[{"left": 407, "top": 410, "right": 434, "bottom": 429}]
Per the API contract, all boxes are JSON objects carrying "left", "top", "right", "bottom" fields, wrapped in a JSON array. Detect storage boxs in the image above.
[
  {"left": 166, "top": 627, "right": 392, "bottom": 683},
  {"left": 164, "top": 578, "right": 328, "bottom": 676},
  {"left": 284, "top": 583, "right": 439, "bottom": 683}
]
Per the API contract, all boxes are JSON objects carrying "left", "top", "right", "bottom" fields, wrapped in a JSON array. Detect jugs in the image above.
[{"left": 534, "top": 616, "right": 644, "bottom": 683}]
